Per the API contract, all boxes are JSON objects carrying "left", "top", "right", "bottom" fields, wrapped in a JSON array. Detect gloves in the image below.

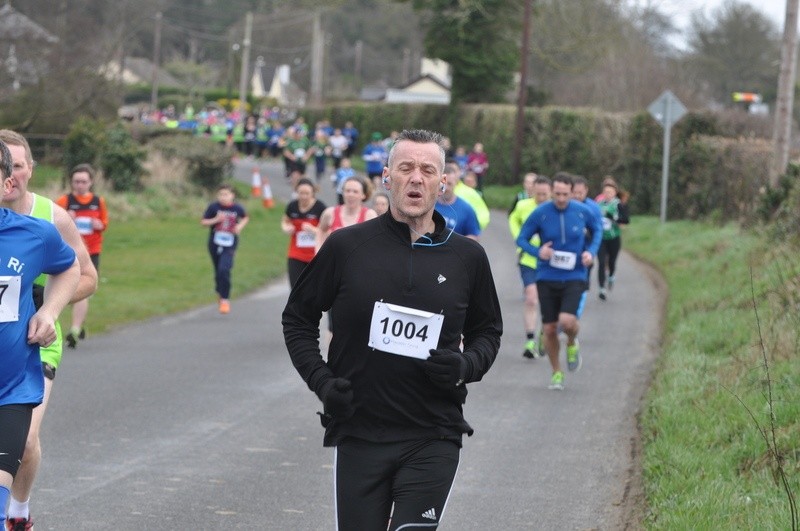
[
  {"left": 425, "top": 349, "right": 467, "bottom": 390},
  {"left": 320, "top": 378, "right": 355, "bottom": 421}
]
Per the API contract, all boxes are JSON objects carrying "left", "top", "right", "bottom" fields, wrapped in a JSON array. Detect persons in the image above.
[
  {"left": 0, "top": 130, "right": 98, "bottom": 531},
  {"left": 0, "top": 142, "right": 80, "bottom": 531},
  {"left": 56, "top": 163, "right": 108, "bottom": 348},
  {"left": 201, "top": 185, "right": 249, "bottom": 314},
  {"left": 282, "top": 129, "right": 505, "bottom": 531},
  {"left": 516, "top": 171, "right": 604, "bottom": 392},
  {"left": 137, "top": 100, "right": 632, "bottom": 360}
]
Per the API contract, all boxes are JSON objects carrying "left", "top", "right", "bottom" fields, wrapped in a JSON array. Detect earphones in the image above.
[
  {"left": 440, "top": 183, "right": 446, "bottom": 192},
  {"left": 382, "top": 177, "right": 387, "bottom": 183}
]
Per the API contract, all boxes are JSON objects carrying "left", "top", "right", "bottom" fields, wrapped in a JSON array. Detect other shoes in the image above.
[
  {"left": 218, "top": 300, "right": 230, "bottom": 314},
  {"left": 599, "top": 289, "right": 607, "bottom": 300},
  {"left": 77, "top": 327, "right": 87, "bottom": 340},
  {"left": 608, "top": 277, "right": 614, "bottom": 288},
  {"left": 523, "top": 342, "right": 536, "bottom": 359},
  {"left": 538, "top": 337, "right": 546, "bottom": 355},
  {"left": 67, "top": 332, "right": 78, "bottom": 349}
]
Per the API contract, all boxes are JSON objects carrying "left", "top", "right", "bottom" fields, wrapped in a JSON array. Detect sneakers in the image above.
[
  {"left": 548, "top": 371, "right": 564, "bottom": 391},
  {"left": 566, "top": 339, "right": 580, "bottom": 373},
  {"left": 5, "top": 511, "right": 34, "bottom": 531}
]
